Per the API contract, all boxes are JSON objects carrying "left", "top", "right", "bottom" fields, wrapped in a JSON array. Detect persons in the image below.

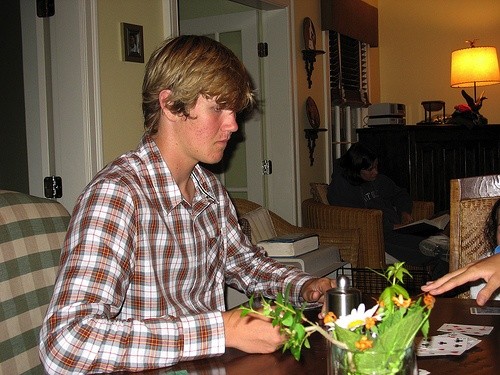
[
  {"left": 419, "top": 194, "right": 500, "bottom": 309},
  {"left": 326, "top": 142, "right": 451, "bottom": 281},
  {"left": 40, "top": 33, "right": 347, "bottom": 375}
]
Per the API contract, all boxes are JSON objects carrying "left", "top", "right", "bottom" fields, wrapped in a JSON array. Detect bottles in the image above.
[{"left": 325, "top": 275, "right": 361, "bottom": 320}]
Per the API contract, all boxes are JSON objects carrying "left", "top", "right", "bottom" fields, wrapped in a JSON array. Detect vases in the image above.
[{"left": 326, "top": 339, "right": 419, "bottom": 375}]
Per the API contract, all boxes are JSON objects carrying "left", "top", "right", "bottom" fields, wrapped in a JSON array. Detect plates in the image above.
[{"left": 306, "top": 96, "right": 320, "bottom": 128}]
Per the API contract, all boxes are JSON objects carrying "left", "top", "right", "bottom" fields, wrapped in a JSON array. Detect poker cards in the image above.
[
  {"left": 413, "top": 322, "right": 494, "bottom": 356},
  {"left": 470, "top": 305, "right": 500, "bottom": 316}
]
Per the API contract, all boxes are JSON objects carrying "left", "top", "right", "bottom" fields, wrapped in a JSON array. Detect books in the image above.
[
  {"left": 256, "top": 234, "right": 319, "bottom": 257},
  {"left": 393, "top": 214, "right": 450, "bottom": 236}
]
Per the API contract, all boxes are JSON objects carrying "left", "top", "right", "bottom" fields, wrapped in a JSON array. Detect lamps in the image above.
[{"left": 450, "top": 39, "right": 500, "bottom": 127}]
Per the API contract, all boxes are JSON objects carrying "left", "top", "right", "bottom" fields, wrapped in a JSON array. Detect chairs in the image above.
[
  {"left": 231, "top": 197, "right": 360, "bottom": 286},
  {"left": 449, "top": 174, "right": 500, "bottom": 274},
  {"left": 302, "top": 198, "right": 434, "bottom": 294},
  {"left": 0, "top": 189, "right": 71, "bottom": 375}
]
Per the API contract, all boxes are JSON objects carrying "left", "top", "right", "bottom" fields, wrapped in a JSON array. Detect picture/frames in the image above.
[{"left": 120, "top": 22, "right": 144, "bottom": 64}]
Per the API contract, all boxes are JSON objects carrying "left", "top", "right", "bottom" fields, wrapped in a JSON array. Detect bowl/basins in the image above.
[{"left": 422, "top": 100, "right": 445, "bottom": 111}]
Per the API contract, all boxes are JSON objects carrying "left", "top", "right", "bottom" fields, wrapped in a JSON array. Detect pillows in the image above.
[
  {"left": 309, "top": 183, "right": 329, "bottom": 205},
  {"left": 238, "top": 207, "right": 277, "bottom": 245}
]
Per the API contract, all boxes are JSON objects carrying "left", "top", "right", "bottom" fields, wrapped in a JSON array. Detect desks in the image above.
[
  {"left": 356, "top": 124, "right": 500, "bottom": 226},
  {"left": 115, "top": 297, "right": 500, "bottom": 375}
]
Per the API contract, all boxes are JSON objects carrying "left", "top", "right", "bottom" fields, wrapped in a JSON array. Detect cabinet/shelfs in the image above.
[{"left": 301, "top": 48, "right": 328, "bottom": 166}]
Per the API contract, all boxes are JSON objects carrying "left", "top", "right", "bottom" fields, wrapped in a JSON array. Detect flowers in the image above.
[{"left": 227, "top": 261, "right": 433, "bottom": 375}]
